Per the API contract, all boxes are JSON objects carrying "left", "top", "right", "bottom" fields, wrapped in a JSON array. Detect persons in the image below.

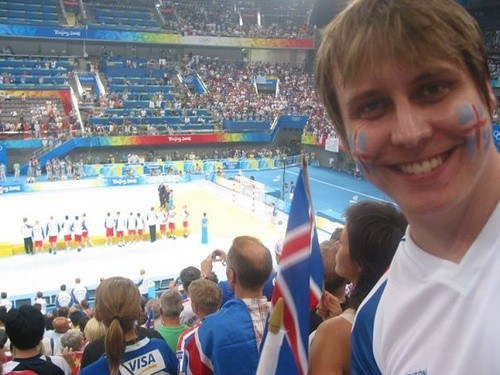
[
  {"left": 12, "top": 8, "right": 359, "bottom": 208},
  {"left": 483, "top": 20, "right": 500, "bottom": 153},
  {"left": 314, "top": 2, "right": 500, "bottom": 373},
  {"left": 0, "top": 189, "right": 405, "bottom": 375}
]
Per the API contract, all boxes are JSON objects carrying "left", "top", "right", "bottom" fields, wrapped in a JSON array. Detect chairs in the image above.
[
  {"left": 0, "top": 275, "right": 191, "bottom": 328},
  {"left": 1, "top": 0, "right": 220, "bottom": 133}
]
